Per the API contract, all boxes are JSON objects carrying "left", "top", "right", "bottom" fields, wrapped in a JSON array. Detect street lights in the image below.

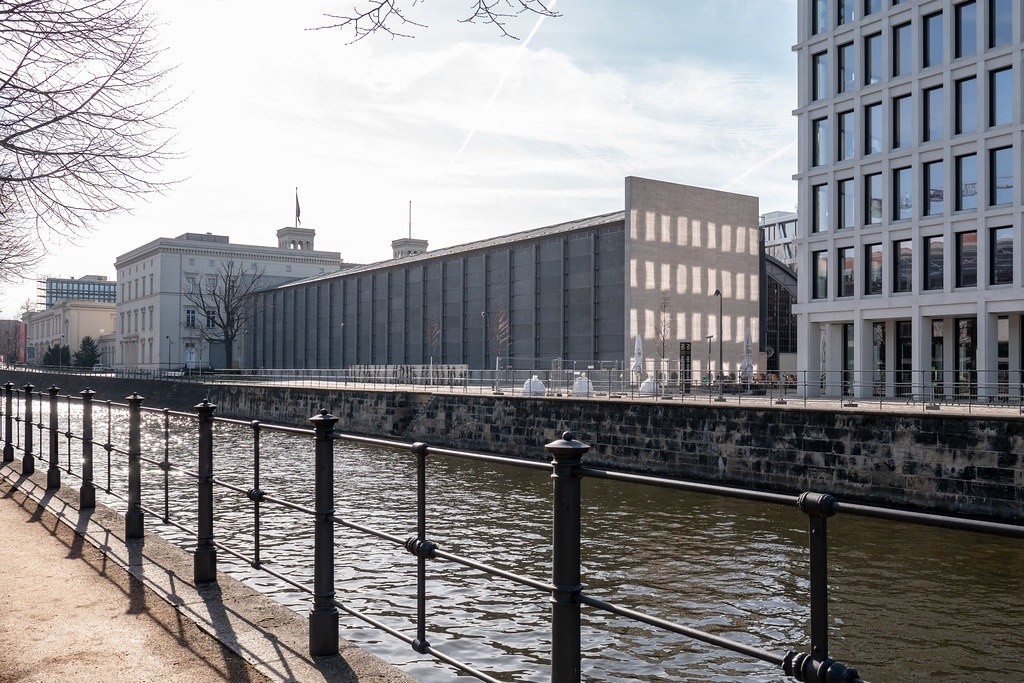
[
  {"left": 713, "top": 289, "right": 726, "bottom": 402},
  {"left": 5, "top": 312, "right": 496, "bottom": 392}
]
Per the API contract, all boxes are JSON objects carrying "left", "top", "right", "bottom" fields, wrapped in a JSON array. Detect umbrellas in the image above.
[
  {"left": 632, "top": 334, "right": 644, "bottom": 390},
  {"left": 739, "top": 327, "right": 753, "bottom": 390}
]
